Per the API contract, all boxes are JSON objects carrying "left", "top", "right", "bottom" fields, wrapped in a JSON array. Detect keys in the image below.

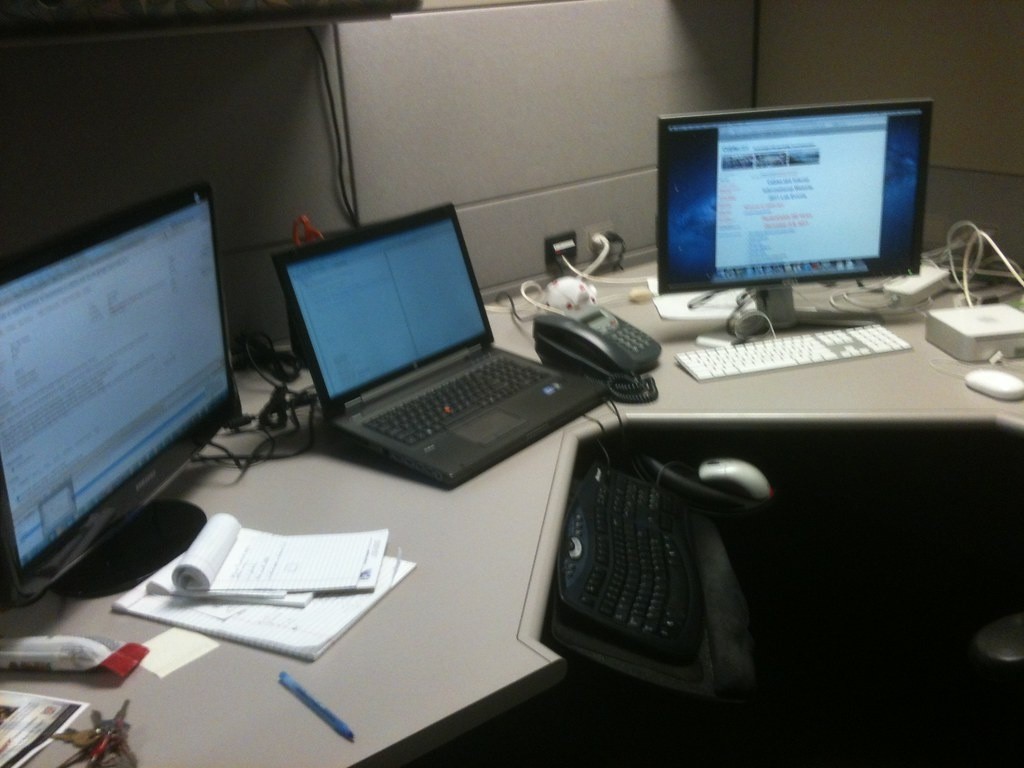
[{"left": 46, "top": 699, "right": 139, "bottom": 768}]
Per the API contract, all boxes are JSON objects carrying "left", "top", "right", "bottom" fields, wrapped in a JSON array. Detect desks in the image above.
[{"left": 0, "top": 258, "right": 1024, "bottom": 768}]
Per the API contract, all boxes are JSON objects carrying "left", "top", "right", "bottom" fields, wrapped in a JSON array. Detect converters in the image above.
[{"left": 882, "top": 266, "right": 950, "bottom": 308}]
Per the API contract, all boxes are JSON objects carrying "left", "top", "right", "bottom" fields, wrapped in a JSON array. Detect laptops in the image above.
[{"left": 270, "top": 203, "right": 612, "bottom": 491}]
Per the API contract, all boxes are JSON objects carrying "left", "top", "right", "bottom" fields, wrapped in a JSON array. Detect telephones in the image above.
[{"left": 532, "top": 302, "right": 663, "bottom": 378}]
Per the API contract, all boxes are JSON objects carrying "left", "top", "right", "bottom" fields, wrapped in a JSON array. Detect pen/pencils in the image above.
[
  {"left": 688, "top": 289, "right": 719, "bottom": 307},
  {"left": 280, "top": 672, "right": 355, "bottom": 740}
]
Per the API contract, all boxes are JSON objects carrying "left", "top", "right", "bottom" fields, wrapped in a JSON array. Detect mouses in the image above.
[
  {"left": 699, "top": 457, "right": 773, "bottom": 500},
  {"left": 965, "top": 370, "right": 1024, "bottom": 398}
]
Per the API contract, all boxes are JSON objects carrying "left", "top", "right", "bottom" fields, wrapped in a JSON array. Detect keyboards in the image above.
[
  {"left": 675, "top": 325, "right": 912, "bottom": 382},
  {"left": 554, "top": 464, "right": 704, "bottom": 662}
]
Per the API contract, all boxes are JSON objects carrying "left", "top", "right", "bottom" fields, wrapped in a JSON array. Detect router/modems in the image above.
[{"left": 926, "top": 302, "right": 1024, "bottom": 363}]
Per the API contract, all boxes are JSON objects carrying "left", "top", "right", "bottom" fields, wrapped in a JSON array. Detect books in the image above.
[{"left": 112, "top": 512, "right": 418, "bottom": 664}]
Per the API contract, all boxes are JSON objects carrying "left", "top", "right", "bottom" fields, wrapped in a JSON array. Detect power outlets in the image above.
[
  {"left": 543, "top": 231, "right": 577, "bottom": 272},
  {"left": 581, "top": 220, "right": 618, "bottom": 260}
]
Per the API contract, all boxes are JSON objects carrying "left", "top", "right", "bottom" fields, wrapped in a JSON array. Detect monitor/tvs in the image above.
[
  {"left": 657, "top": 100, "right": 933, "bottom": 345},
  {"left": 0, "top": 182, "right": 238, "bottom": 609}
]
